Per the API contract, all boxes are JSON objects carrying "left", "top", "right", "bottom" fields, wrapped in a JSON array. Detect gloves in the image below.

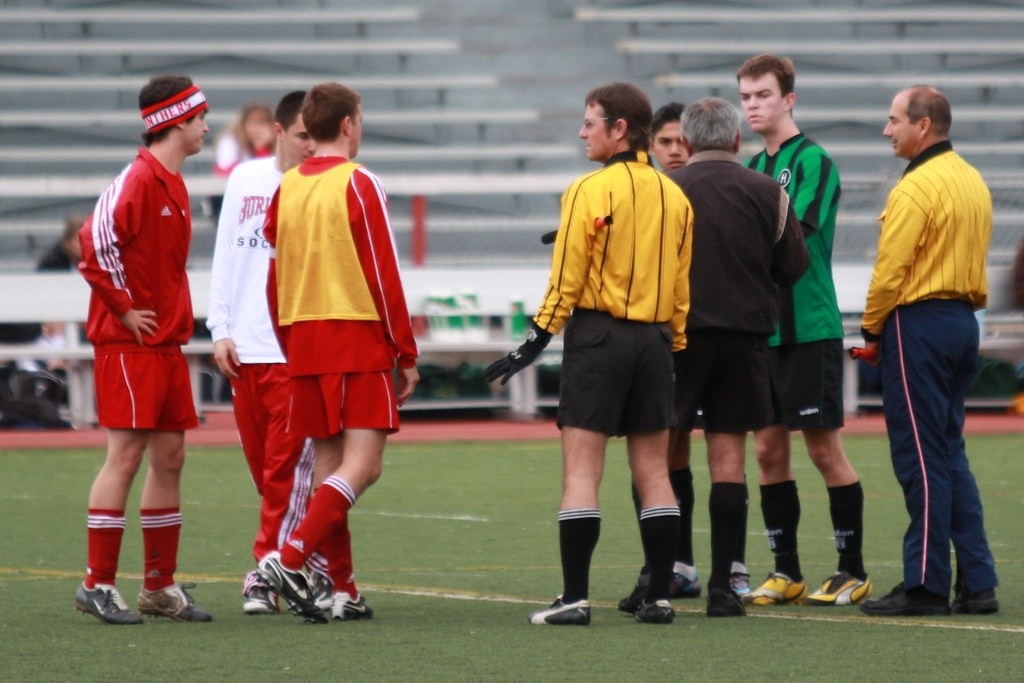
[{"left": 482, "top": 338, "right": 543, "bottom": 385}]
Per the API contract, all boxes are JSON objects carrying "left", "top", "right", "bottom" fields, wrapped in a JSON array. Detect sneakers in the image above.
[
  {"left": 243, "top": 570, "right": 281, "bottom": 614},
  {"left": 137, "top": 581, "right": 212, "bottom": 622},
  {"left": 74, "top": 581, "right": 142, "bottom": 624},
  {"left": 634, "top": 598, "right": 674, "bottom": 623},
  {"left": 860, "top": 581, "right": 950, "bottom": 616},
  {"left": 744, "top": 572, "right": 808, "bottom": 606},
  {"left": 706, "top": 588, "right": 746, "bottom": 617},
  {"left": 290, "top": 572, "right": 333, "bottom": 614},
  {"left": 669, "top": 572, "right": 702, "bottom": 598},
  {"left": 730, "top": 572, "right": 750, "bottom": 600},
  {"left": 528, "top": 594, "right": 590, "bottom": 626},
  {"left": 620, "top": 585, "right": 645, "bottom": 611},
  {"left": 331, "top": 592, "right": 373, "bottom": 620},
  {"left": 951, "top": 582, "right": 998, "bottom": 613},
  {"left": 806, "top": 573, "right": 873, "bottom": 606},
  {"left": 257, "top": 550, "right": 328, "bottom": 623}
]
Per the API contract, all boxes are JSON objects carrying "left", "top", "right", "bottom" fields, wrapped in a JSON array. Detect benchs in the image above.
[{"left": 0, "top": 0, "right": 1024, "bottom": 426}]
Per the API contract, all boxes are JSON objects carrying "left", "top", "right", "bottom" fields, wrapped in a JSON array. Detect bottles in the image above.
[{"left": 510, "top": 302, "right": 526, "bottom": 339}]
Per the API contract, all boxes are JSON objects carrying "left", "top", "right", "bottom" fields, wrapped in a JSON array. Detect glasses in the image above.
[{"left": 585, "top": 117, "right": 617, "bottom": 128}]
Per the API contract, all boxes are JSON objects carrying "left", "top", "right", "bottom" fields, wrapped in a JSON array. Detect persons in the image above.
[
  {"left": 205, "top": 83, "right": 419, "bottom": 623},
  {"left": 404, "top": 346, "right": 507, "bottom": 416},
  {"left": 77, "top": 77, "right": 214, "bottom": 625},
  {"left": 31, "top": 216, "right": 110, "bottom": 393},
  {"left": 481, "top": 84, "right": 693, "bottom": 627},
  {"left": 859, "top": 84, "right": 1002, "bottom": 618},
  {"left": 624, "top": 54, "right": 873, "bottom": 608}
]
